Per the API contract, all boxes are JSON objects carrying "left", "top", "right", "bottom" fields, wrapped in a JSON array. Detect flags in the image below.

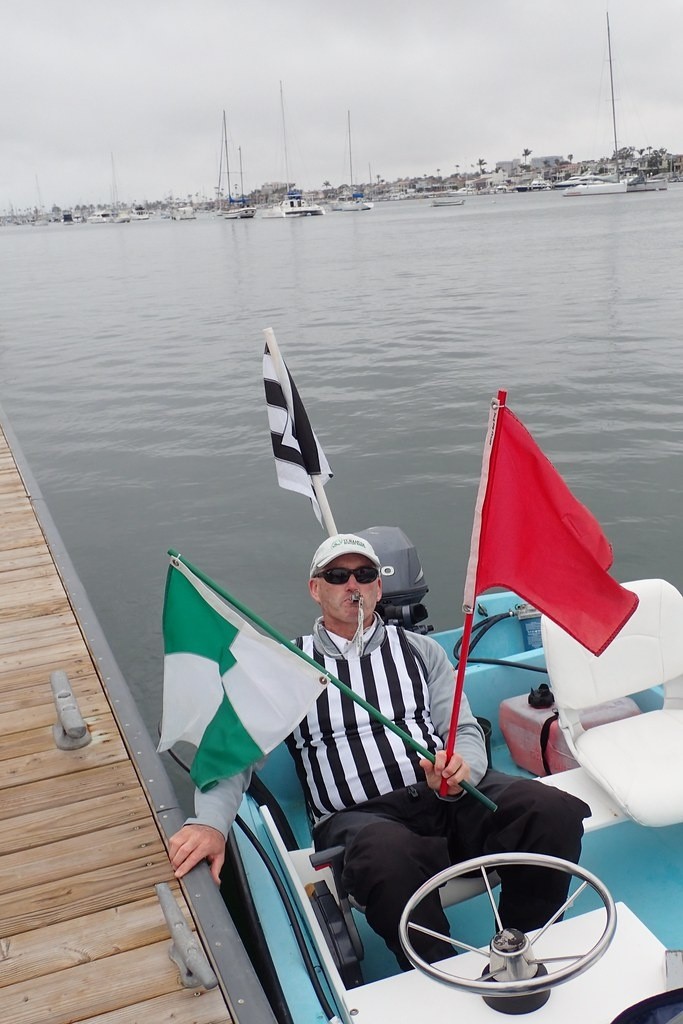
[
  {"left": 156, "top": 556, "right": 331, "bottom": 795},
  {"left": 263, "top": 343, "right": 335, "bottom": 529},
  {"left": 462, "top": 398, "right": 640, "bottom": 656}
]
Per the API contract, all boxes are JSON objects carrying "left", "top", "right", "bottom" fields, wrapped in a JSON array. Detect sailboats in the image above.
[
  {"left": 1, "top": 78, "right": 376, "bottom": 227},
  {"left": 583, "top": 11, "right": 668, "bottom": 192}
]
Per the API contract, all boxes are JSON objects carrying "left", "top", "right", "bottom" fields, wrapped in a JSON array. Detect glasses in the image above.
[{"left": 314, "top": 565, "right": 379, "bottom": 585}]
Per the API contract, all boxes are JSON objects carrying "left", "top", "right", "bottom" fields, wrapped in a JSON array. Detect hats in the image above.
[{"left": 310, "top": 534, "right": 382, "bottom": 578}]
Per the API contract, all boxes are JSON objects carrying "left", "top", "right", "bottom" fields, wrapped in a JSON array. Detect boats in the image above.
[
  {"left": 553, "top": 169, "right": 604, "bottom": 190},
  {"left": 515, "top": 177, "right": 552, "bottom": 192},
  {"left": 227, "top": 524, "right": 683, "bottom": 1024},
  {"left": 430, "top": 200, "right": 465, "bottom": 207},
  {"left": 377, "top": 182, "right": 513, "bottom": 201},
  {"left": 562, "top": 178, "right": 629, "bottom": 196}
]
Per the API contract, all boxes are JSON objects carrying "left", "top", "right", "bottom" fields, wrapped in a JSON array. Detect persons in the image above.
[{"left": 168, "top": 534, "right": 593, "bottom": 971}]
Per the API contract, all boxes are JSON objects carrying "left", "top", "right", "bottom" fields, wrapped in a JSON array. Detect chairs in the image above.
[
  {"left": 306, "top": 836, "right": 502, "bottom": 913},
  {"left": 539, "top": 579, "right": 683, "bottom": 829}
]
